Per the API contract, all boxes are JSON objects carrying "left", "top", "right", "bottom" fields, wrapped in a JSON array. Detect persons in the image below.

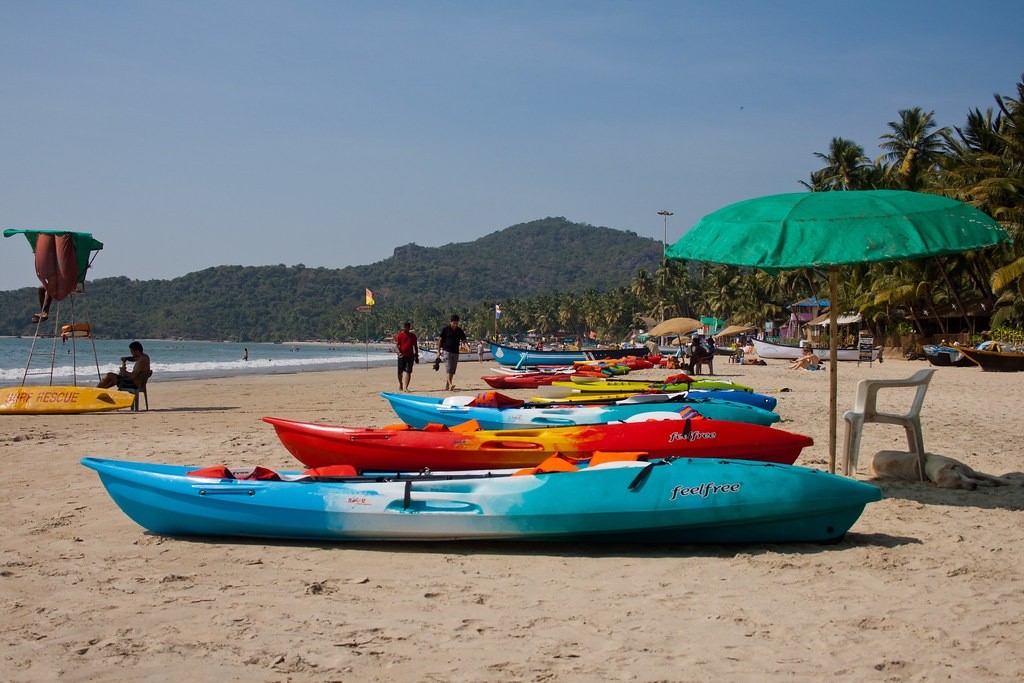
[
  {"left": 437, "top": 315, "right": 471, "bottom": 391},
  {"left": 707, "top": 336, "right": 714, "bottom": 347},
  {"left": 687, "top": 338, "right": 708, "bottom": 375},
  {"left": 97, "top": 342, "right": 150, "bottom": 388},
  {"left": 561, "top": 337, "right": 582, "bottom": 351},
  {"left": 790, "top": 344, "right": 819, "bottom": 370},
  {"left": 243, "top": 348, "right": 248, "bottom": 360},
  {"left": 731, "top": 339, "right": 755, "bottom": 355},
  {"left": 396, "top": 322, "right": 418, "bottom": 392},
  {"left": 527, "top": 342, "right": 544, "bottom": 351},
  {"left": 32, "top": 285, "right": 52, "bottom": 323},
  {"left": 478, "top": 342, "right": 485, "bottom": 363},
  {"left": 940, "top": 340, "right": 947, "bottom": 347}
]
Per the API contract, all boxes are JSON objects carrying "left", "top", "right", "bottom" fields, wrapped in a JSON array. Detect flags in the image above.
[
  {"left": 496, "top": 305, "right": 501, "bottom": 319},
  {"left": 366, "top": 288, "right": 375, "bottom": 306}
]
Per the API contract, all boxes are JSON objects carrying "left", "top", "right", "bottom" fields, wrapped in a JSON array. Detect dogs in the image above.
[{"left": 871, "top": 449, "right": 1024, "bottom": 491}]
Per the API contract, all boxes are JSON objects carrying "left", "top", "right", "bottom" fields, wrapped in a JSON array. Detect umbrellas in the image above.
[
  {"left": 802, "top": 310, "right": 862, "bottom": 336},
  {"left": 672, "top": 336, "right": 690, "bottom": 352},
  {"left": 646, "top": 317, "right": 703, "bottom": 365},
  {"left": 663, "top": 189, "right": 1008, "bottom": 474},
  {"left": 715, "top": 326, "right": 752, "bottom": 339}
]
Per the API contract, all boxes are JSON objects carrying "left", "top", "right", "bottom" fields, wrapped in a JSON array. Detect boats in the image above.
[
  {"left": 749, "top": 337, "right": 883, "bottom": 362},
  {"left": 80, "top": 454, "right": 884, "bottom": 547},
  {"left": 922, "top": 339, "right": 1024, "bottom": 373},
  {"left": 261, "top": 414, "right": 815, "bottom": 473},
  {"left": 479, "top": 339, "right": 778, "bottom": 415},
  {"left": 381, "top": 391, "right": 780, "bottom": 431},
  {"left": 417, "top": 347, "right": 495, "bottom": 363}
]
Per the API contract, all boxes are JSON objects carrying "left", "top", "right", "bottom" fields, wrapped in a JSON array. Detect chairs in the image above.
[
  {"left": 696, "top": 353, "right": 714, "bottom": 375},
  {"left": 729, "top": 349, "right": 744, "bottom": 363},
  {"left": 841, "top": 369, "right": 938, "bottom": 482},
  {"left": 117, "top": 370, "right": 152, "bottom": 412}
]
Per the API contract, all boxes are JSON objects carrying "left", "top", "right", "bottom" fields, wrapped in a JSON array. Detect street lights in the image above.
[{"left": 657, "top": 209, "right": 674, "bottom": 347}]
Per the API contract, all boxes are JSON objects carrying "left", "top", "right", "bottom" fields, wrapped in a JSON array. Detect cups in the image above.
[{"left": 122, "top": 361, "right": 126, "bottom": 368}]
